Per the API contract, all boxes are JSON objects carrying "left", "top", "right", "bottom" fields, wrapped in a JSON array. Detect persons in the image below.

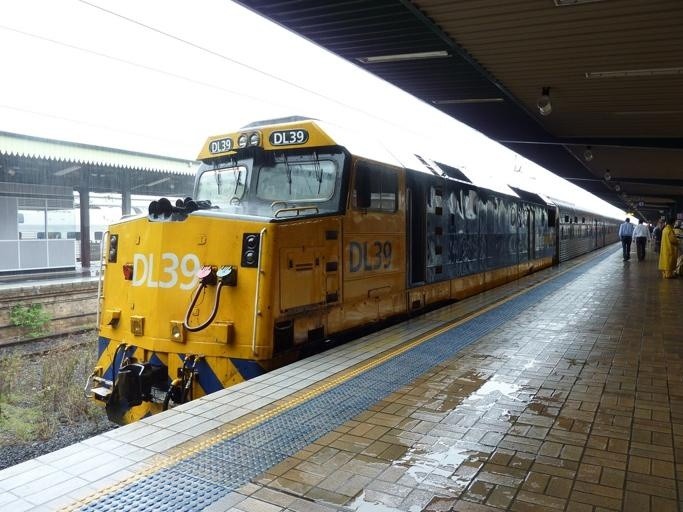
[{"left": 619, "top": 218, "right": 679, "bottom": 279}]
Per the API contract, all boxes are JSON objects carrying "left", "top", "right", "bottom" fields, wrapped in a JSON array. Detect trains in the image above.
[{"left": 82, "top": 114, "right": 636, "bottom": 426}]
